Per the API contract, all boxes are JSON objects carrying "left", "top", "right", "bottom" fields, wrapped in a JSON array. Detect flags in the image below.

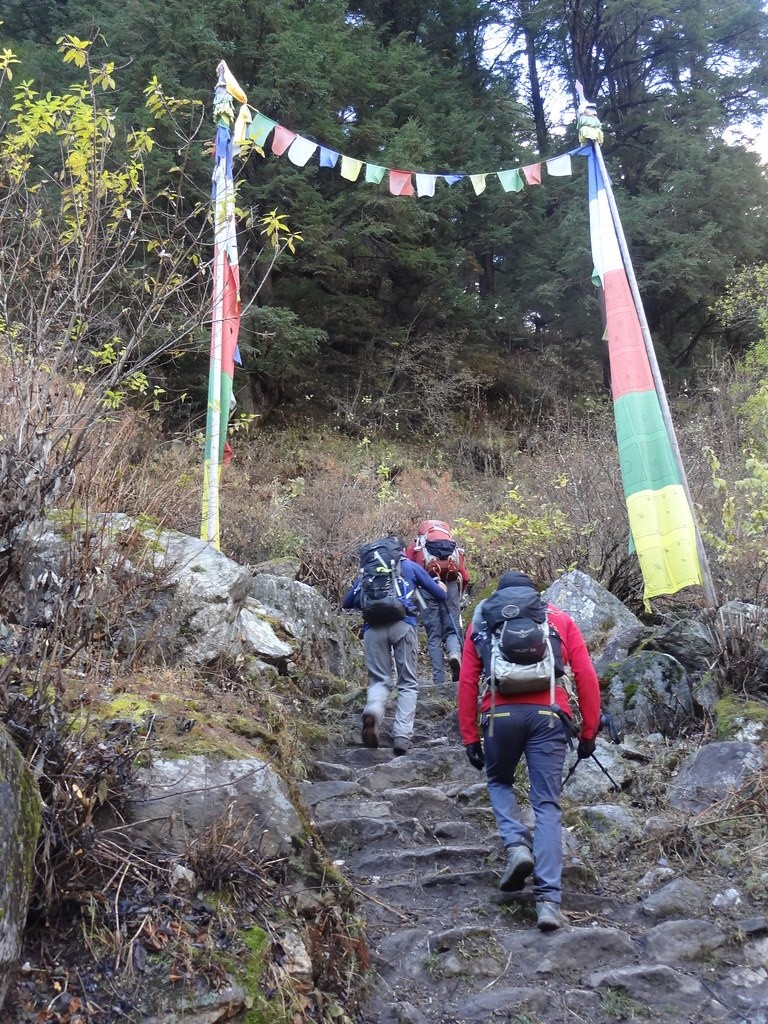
[{"left": 181, "top": 62, "right": 703, "bottom": 614}]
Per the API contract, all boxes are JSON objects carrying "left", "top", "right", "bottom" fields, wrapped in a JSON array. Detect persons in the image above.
[
  {"left": 412, "top": 518, "right": 470, "bottom": 685},
  {"left": 457, "top": 570, "right": 601, "bottom": 928},
  {"left": 341, "top": 535, "right": 447, "bottom": 753}
]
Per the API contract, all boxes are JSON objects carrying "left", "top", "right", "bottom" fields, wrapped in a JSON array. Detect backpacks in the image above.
[
  {"left": 417, "top": 518, "right": 464, "bottom": 585},
  {"left": 359, "top": 535, "right": 411, "bottom": 622},
  {"left": 469, "top": 587, "right": 566, "bottom": 735}
]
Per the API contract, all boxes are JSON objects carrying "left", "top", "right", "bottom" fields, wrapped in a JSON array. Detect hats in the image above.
[{"left": 495, "top": 569, "right": 539, "bottom": 592}]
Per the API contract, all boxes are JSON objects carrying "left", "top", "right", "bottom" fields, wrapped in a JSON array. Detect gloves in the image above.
[
  {"left": 577, "top": 736, "right": 596, "bottom": 759},
  {"left": 467, "top": 741, "right": 489, "bottom": 772}
]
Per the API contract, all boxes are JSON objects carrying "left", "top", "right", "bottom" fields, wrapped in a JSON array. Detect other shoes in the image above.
[
  {"left": 449, "top": 652, "right": 462, "bottom": 681},
  {"left": 498, "top": 846, "right": 535, "bottom": 893},
  {"left": 533, "top": 900, "right": 569, "bottom": 929},
  {"left": 394, "top": 738, "right": 417, "bottom": 751},
  {"left": 362, "top": 710, "right": 384, "bottom": 749}
]
